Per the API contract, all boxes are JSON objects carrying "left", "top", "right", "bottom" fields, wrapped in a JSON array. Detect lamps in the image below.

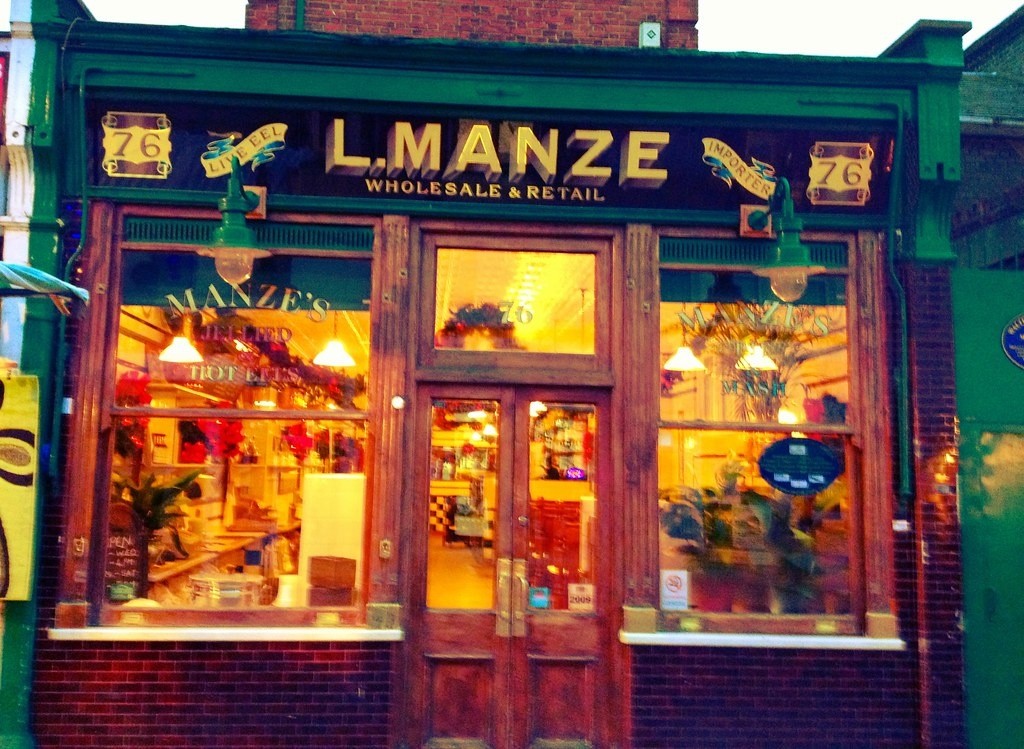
[
  {"left": 157, "top": 307, "right": 204, "bottom": 364},
  {"left": 739, "top": 177, "right": 828, "bottom": 302},
  {"left": 731, "top": 306, "right": 779, "bottom": 374},
  {"left": 663, "top": 303, "right": 705, "bottom": 376},
  {"left": 196, "top": 155, "right": 272, "bottom": 284},
  {"left": 311, "top": 310, "right": 357, "bottom": 373}
]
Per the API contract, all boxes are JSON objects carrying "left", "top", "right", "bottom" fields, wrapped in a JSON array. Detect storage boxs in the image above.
[{"left": 309, "top": 556, "right": 357, "bottom": 609}]
